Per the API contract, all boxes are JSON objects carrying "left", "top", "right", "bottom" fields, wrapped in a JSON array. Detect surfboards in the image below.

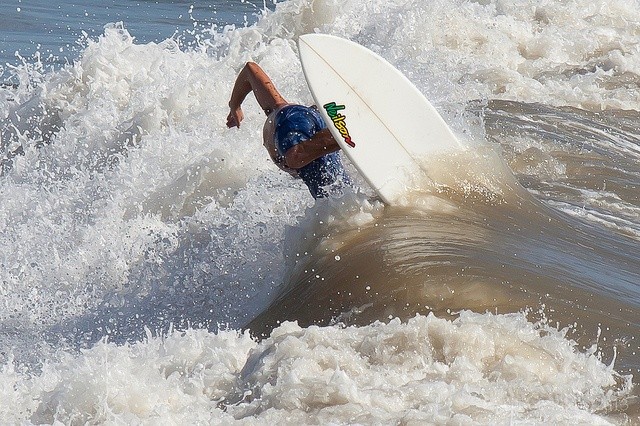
[{"left": 298, "top": 33, "right": 467, "bottom": 208}]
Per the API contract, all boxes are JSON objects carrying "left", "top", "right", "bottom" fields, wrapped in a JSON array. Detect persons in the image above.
[{"left": 225, "top": 62, "right": 349, "bottom": 201}]
